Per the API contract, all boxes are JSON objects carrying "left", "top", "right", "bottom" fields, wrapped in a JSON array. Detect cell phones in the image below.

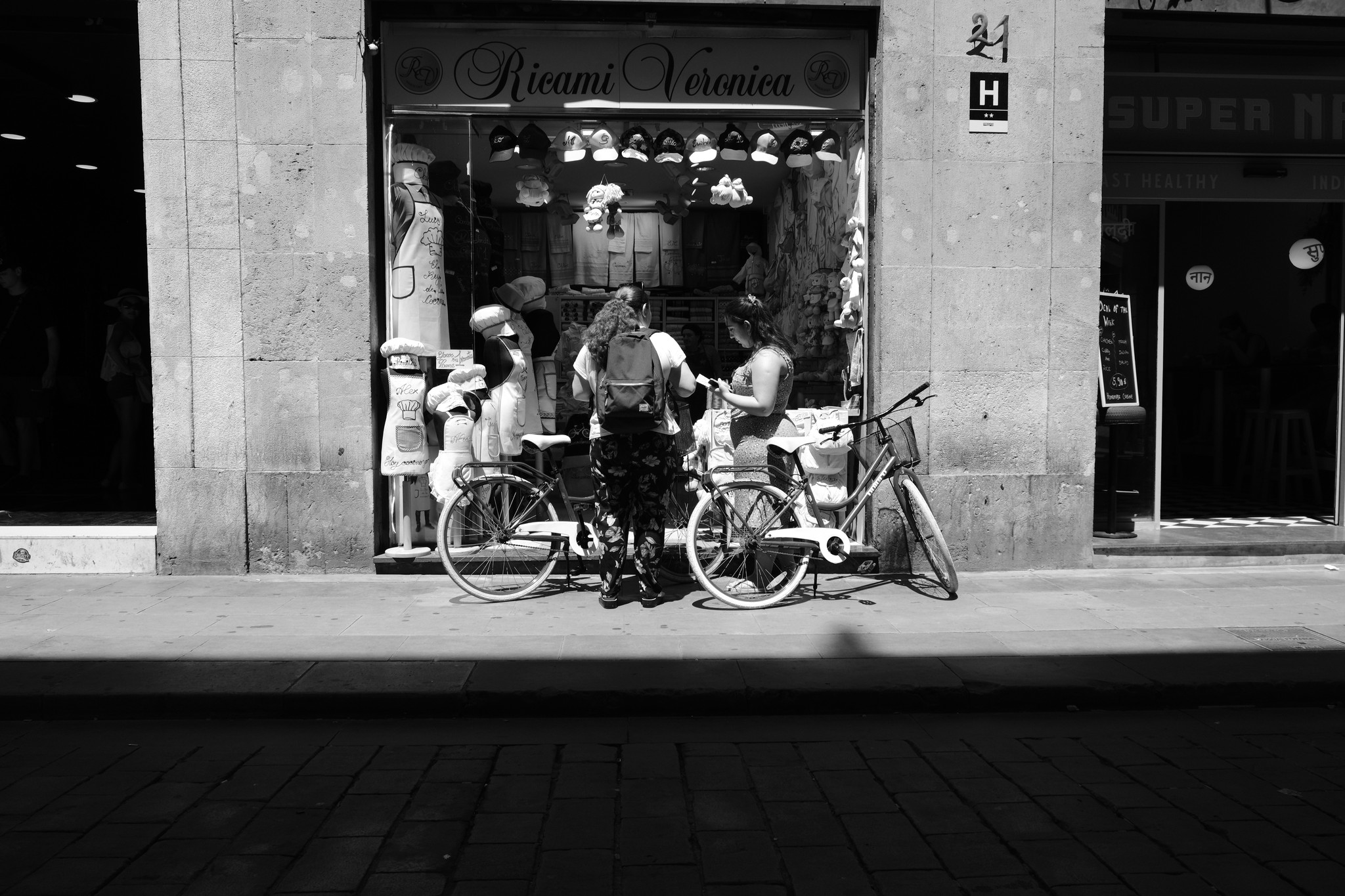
[{"left": 708, "top": 378, "right": 734, "bottom": 394}]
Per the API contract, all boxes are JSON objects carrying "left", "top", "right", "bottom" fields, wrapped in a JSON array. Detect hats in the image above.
[
  {"left": 620, "top": 125, "right": 654, "bottom": 163},
  {"left": 447, "top": 364, "right": 488, "bottom": 393},
  {"left": 518, "top": 123, "right": 552, "bottom": 160},
  {"left": 469, "top": 304, "right": 516, "bottom": 340},
  {"left": 488, "top": 124, "right": 518, "bottom": 163},
  {"left": 750, "top": 128, "right": 782, "bottom": 165},
  {"left": 684, "top": 126, "right": 718, "bottom": 163},
  {"left": 779, "top": 129, "right": 813, "bottom": 168},
  {"left": 813, "top": 128, "right": 842, "bottom": 162},
  {"left": 717, "top": 123, "right": 750, "bottom": 161},
  {"left": 425, "top": 382, "right": 470, "bottom": 412},
  {"left": 511, "top": 276, "right": 547, "bottom": 318},
  {"left": 652, "top": 128, "right": 686, "bottom": 163},
  {"left": 551, "top": 126, "right": 589, "bottom": 163},
  {"left": 380, "top": 337, "right": 425, "bottom": 370},
  {"left": 493, "top": 283, "right": 526, "bottom": 320},
  {"left": 391, "top": 142, "right": 493, "bottom": 211},
  {"left": 587, "top": 123, "right": 620, "bottom": 161}
]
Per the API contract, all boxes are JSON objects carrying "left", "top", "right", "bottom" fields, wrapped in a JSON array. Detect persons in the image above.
[
  {"left": 732, "top": 243, "right": 769, "bottom": 294},
  {"left": 380, "top": 144, "right": 591, "bottom": 508},
  {"left": 571, "top": 285, "right": 697, "bottom": 609},
  {"left": 708, "top": 296, "right": 793, "bottom": 593},
  {"left": 680, "top": 322, "right": 724, "bottom": 425}
]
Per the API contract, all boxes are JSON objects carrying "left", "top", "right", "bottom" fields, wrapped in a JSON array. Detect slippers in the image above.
[
  {"left": 726, "top": 576, "right": 775, "bottom": 593},
  {"left": 781, "top": 567, "right": 796, "bottom": 579}
]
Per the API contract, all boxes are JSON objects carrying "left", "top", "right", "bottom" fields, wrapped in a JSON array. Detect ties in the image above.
[{"left": 421, "top": 186, "right": 431, "bottom": 203}]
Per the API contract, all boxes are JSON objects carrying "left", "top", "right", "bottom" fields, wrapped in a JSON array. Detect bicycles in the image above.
[
  {"left": 568, "top": 423, "right": 590, "bottom": 438},
  {"left": 437, "top": 401, "right": 731, "bottom": 603},
  {"left": 685, "top": 382, "right": 959, "bottom": 610}
]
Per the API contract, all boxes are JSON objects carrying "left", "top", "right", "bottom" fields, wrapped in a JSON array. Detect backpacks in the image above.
[
  {"left": 594, "top": 325, "right": 671, "bottom": 435},
  {"left": 563, "top": 411, "right": 591, "bottom": 456}
]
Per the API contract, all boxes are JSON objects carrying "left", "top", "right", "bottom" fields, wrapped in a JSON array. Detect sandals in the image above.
[
  {"left": 642, "top": 591, "right": 666, "bottom": 608},
  {"left": 598, "top": 592, "right": 619, "bottom": 609}
]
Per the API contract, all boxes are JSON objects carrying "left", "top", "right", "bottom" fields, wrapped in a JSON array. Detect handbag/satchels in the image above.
[
  {"left": 777, "top": 226, "right": 795, "bottom": 253},
  {"left": 668, "top": 400, "right": 697, "bottom": 458},
  {"left": 790, "top": 410, "right": 854, "bottom": 532}
]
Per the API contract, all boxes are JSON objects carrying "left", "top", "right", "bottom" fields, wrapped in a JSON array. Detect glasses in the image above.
[{"left": 617, "top": 281, "right": 645, "bottom": 311}]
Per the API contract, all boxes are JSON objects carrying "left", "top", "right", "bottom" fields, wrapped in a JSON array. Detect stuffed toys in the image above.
[{"left": 516, "top": 173, "right": 865, "bottom": 381}]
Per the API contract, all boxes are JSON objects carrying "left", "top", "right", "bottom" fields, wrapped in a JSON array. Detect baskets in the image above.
[{"left": 847, "top": 416, "right": 921, "bottom": 480}]
